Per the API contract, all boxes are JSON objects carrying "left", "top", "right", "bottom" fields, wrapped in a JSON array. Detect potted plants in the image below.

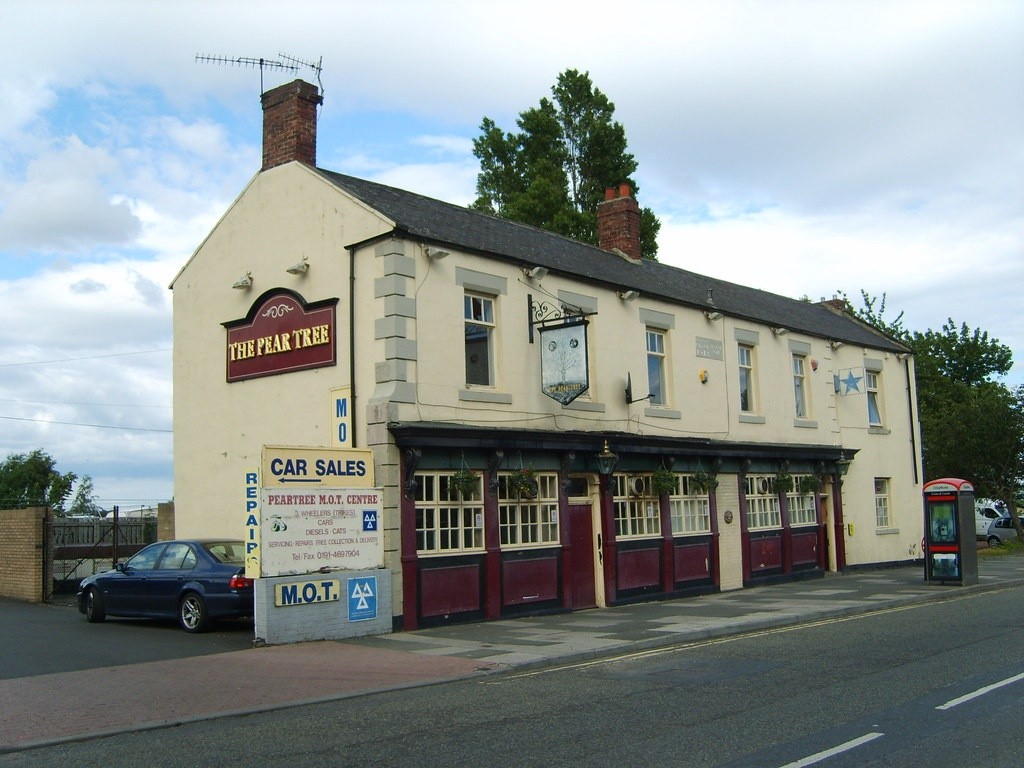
[
  {"left": 652, "top": 470, "right": 680, "bottom": 496},
  {"left": 688, "top": 470, "right": 720, "bottom": 495},
  {"left": 800, "top": 476, "right": 820, "bottom": 494},
  {"left": 772, "top": 471, "right": 794, "bottom": 494}
]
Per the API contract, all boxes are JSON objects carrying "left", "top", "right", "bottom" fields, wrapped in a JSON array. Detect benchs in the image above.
[{"left": 158, "top": 558, "right": 194, "bottom": 569}]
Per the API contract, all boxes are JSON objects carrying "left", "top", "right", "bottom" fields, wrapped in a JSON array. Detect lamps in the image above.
[
  {"left": 896, "top": 353, "right": 911, "bottom": 360},
  {"left": 232, "top": 276, "right": 253, "bottom": 290},
  {"left": 771, "top": 327, "right": 789, "bottom": 337},
  {"left": 285, "top": 262, "right": 310, "bottom": 275},
  {"left": 524, "top": 267, "right": 549, "bottom": 281},
  {"left": 834, "top": 450, "right": 851, "bottom": 490},
  {"left": 703, "top": 311, "right": 723, "bottom": 321},
  {"left": 616, "top": 290, "right": 640, "bottom": 302},
  {"left": 830, "top": 341, "right": 846, "bottom": 350},
  {"left": 425, "top": 248, "right": 450, "bottom": 260},
  {"left": 596, "top": 439, "right": 620, "bottom": 494}
]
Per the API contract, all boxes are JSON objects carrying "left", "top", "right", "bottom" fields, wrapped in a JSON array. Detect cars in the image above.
[
  {"left": 986, "top": 515, "right": 1024, "bottom": 550},
  {"left": 76, "top": 537, "right": 254, "bottom": 634}
]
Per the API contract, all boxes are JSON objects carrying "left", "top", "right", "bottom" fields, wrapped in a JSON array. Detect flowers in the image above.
[
  {"left": 444, "top": 469, "right": 481, "bottom": 499},
  {"left": 507, "top": 464, "right": 538, "bottom": 500}
]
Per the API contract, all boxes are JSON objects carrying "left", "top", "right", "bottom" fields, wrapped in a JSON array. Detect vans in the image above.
[{"left": 975, "top": 506, "right": 1004, "bottom": 541}]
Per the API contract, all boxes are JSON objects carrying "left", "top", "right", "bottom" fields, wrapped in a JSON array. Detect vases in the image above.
[
  {"left": 457, "top": 479, "right": 474, "bottom": 486},
  {"left": 516, "top": 478, "right": 532, "bottom": 487}
]
